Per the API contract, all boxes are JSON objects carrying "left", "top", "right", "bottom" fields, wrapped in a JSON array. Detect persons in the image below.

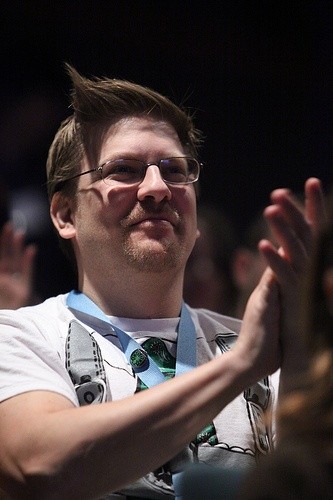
[
  {"left": 0, "top": 189, "right": 332, "bottom": 311},
  {"left": 0, "top": 62, "right": 333, "bottom": 500}
]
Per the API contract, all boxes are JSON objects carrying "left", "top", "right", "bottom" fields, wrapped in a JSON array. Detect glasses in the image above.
[{"left": 54, "top": 156, "right": 204, "bottom": 192}]
